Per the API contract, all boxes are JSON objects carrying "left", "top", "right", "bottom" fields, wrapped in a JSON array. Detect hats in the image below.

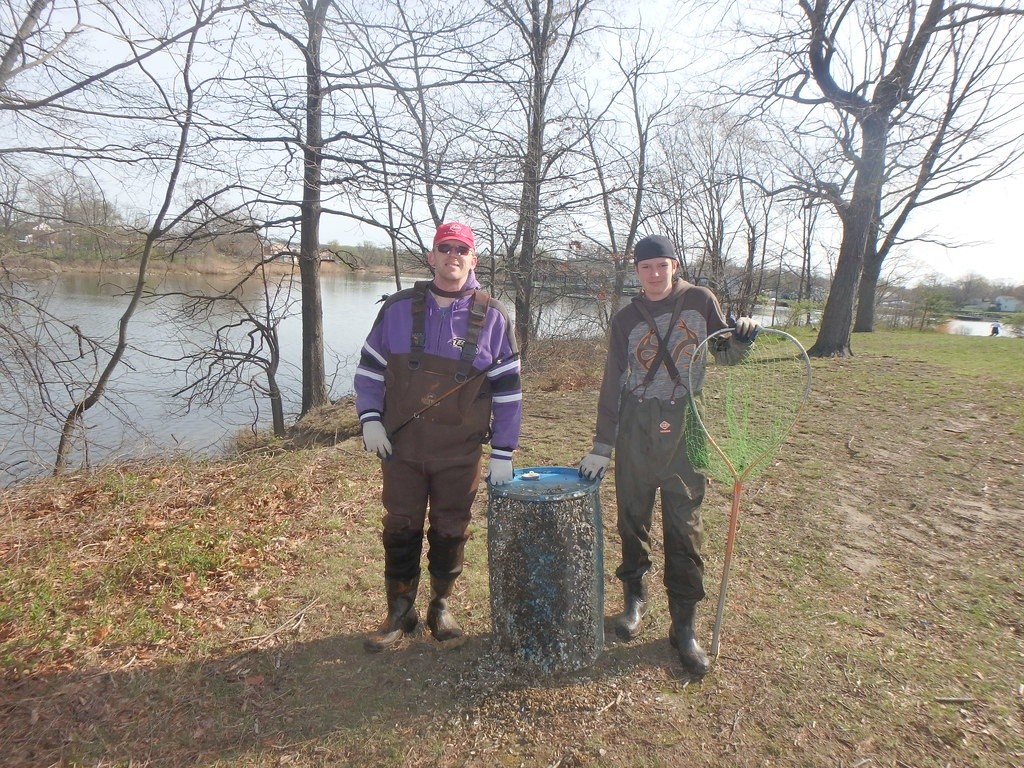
[
  {"left": 634, "top": 234, "right": 676, "bottom": 262},
  {"left": 433, "top": 223, "right": 475, "bottom": 251}
]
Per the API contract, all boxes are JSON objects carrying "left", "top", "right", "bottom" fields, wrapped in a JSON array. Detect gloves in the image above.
[
  {"left": 362, "top": 421, "right": 392, "bottom": 458},
  {"left": 578, "top": 441, "right": 611, "bottom": 481},
  {"left": 488, "top": 457, "right": 514, "bottom": 485},
  {"left": 726, "top": 315, "right": 759, "bottom": 343}
]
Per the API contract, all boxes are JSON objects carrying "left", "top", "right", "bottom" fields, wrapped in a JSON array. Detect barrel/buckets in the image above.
[{"left": 486, "top": 466, "right": 605, "bottom": 676}]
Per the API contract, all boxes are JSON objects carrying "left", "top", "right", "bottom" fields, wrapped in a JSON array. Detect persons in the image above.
[
  {"left": 990, "top": 320, "right": 1003, "bottom": 336},
  {"left": 576, "top": 236, "right": 761, "bottom": 675},
  {"left": 354, "top": 222, "right": 523, "bottom": 653}
]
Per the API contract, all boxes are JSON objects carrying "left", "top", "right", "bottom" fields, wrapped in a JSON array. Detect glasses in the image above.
[{"left": 435, "top": 243, "right": 472, "bottom": 255}]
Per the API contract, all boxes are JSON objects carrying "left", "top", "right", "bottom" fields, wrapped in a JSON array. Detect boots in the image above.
[
  {"left": 364, "top": 528, "right": 421, "bottom": 652},
  {"left": 426, "top": 527, "right": 463, "bottom": 641},
  {"left": 669, "top": 594, "right": 709, "bottom": 674},
  {"left": 617, "top": 575, "right": 649, "bottom": 637}
]
